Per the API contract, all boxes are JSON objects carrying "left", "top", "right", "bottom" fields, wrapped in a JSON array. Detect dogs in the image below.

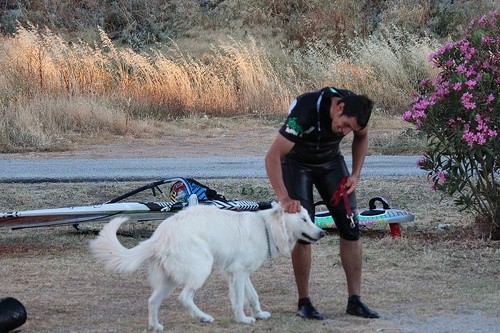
[{"left": 87, "top": 202, "right": 327, "bottom": 332}]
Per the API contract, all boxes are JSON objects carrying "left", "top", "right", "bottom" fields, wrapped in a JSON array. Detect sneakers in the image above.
[
  {"left": 346, "top": 295, "right": 378, "bottom": 318},
  {"left": 296, "top": 297, "right": 324, "bottom": 319}
]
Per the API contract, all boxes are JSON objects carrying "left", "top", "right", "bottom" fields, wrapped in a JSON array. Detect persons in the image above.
[{"left": 265, "top": 86, "right": 381, "bottom": 320}]
